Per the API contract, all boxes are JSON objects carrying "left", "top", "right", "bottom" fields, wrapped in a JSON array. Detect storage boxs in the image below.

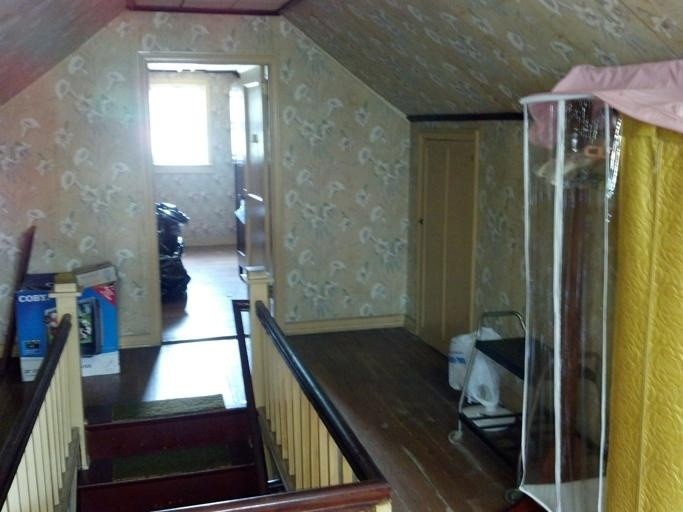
[{"left": 16, "top": 272, "right": 120, "bottom": 382}]
